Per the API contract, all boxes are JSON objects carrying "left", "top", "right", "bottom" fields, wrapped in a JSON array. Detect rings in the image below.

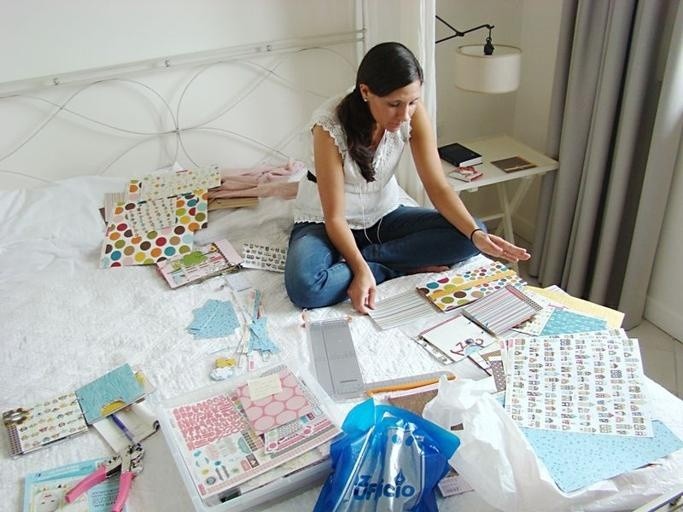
[{"left": 112, "top": 414, "right": 135, "bottom": 441}]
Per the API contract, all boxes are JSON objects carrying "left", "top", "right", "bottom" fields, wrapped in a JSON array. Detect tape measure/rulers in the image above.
[{"left": 65, "top": 442, "right": 144, "bottom": 512}]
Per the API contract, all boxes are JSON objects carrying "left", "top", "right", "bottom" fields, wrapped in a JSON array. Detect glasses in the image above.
[{"left": 434, "top": 9, "right": 524, "bottom": 96}]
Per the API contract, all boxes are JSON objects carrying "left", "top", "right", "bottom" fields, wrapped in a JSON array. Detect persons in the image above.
[{"left": 284, "top": 42, "right": 532, "bottom": 315}]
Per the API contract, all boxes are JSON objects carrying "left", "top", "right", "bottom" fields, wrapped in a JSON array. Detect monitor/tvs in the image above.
[{"left": 438, "top": 143, "right": 483, "bottom": 169}]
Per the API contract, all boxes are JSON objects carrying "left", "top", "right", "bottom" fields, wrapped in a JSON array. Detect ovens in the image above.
[{"left": 1, "top": 25, "right": 683, "bottom": 512}]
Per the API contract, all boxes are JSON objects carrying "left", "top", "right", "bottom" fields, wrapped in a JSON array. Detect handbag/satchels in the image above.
[{"left": 470, "top": 229, "right": 481, "bottom": 242}]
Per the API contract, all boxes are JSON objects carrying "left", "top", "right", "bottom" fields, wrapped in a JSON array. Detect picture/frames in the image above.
[{"left": 435, "top": 133, "right": 559, "bottom": 275}]
